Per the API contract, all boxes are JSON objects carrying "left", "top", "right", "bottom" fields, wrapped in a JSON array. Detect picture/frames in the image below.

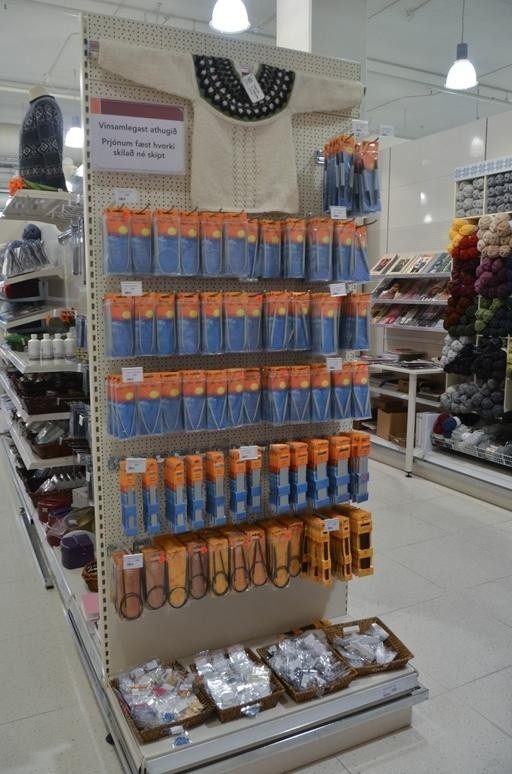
[{"left": 369, "top": 251, "right": 450, "bottom": 330}]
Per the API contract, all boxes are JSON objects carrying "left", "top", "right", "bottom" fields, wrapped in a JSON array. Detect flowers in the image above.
[{"left": 7, "top": 176, "right": 59, "bottom": 197}]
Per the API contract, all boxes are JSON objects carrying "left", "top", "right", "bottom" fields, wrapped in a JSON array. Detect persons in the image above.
[{"left": 18, "top": 81, "right": 67, "bottom": 192}]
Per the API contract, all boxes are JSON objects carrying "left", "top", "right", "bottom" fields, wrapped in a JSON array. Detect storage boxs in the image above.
[{"left": 355, "top": 394, "right": 417, "bottom": 442}]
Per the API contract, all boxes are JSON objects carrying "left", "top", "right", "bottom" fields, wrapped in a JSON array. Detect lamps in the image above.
[
  {"left": 203, "top": 0, "right": 255, "bottom": 38},
  {"left": 438, "top": 0, "right": 481, "bottom": 94}
]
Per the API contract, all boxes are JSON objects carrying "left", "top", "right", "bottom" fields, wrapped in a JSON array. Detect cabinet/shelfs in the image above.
[
  {"left": 0, "top": 186, "right": 103, "bottom": 695},
  {"left": 354, "top": 350, "right": 445, "bottom": 483},
  {"left": 433, "top": 169, "right": 512, "bottom": 508},
  {"left": 368, "top": 246, "right": 452, "bottom": 334}
]
